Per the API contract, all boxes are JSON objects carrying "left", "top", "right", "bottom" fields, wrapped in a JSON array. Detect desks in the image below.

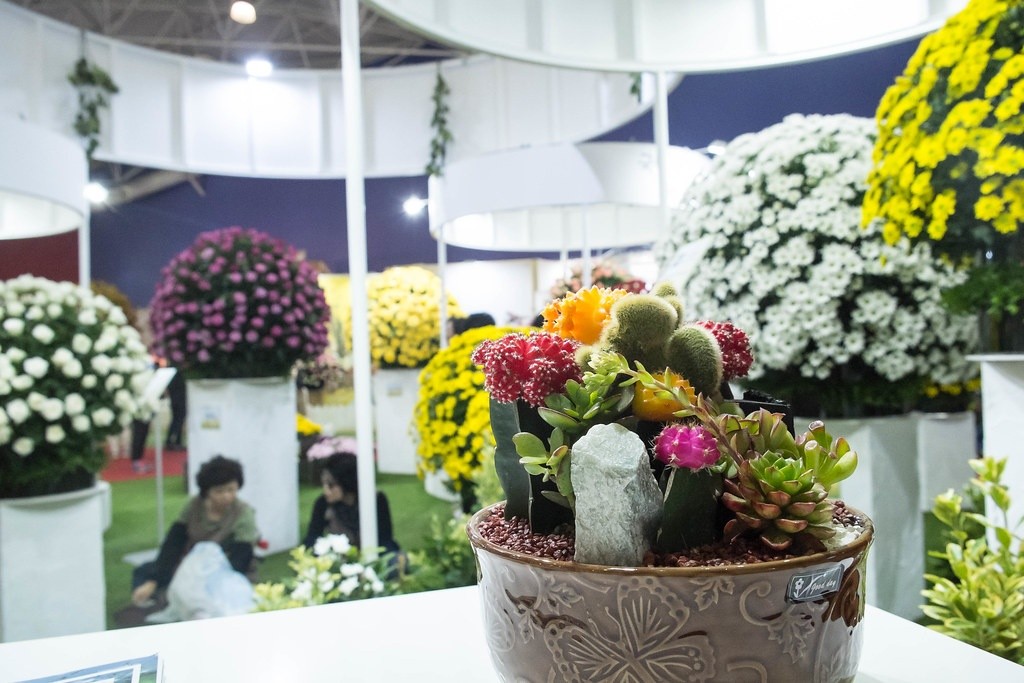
[{"left": 0, "top": 584, "right": 1024, "bottom": 683}]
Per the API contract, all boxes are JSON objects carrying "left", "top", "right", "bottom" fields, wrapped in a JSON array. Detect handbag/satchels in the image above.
[{"left": 143, "top": 540, "right": 263, "bottom": 626}]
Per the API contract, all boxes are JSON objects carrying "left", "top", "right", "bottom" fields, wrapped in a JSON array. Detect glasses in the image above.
[{"left": 322, "top": 479, "right": 339, "bottom": 488}]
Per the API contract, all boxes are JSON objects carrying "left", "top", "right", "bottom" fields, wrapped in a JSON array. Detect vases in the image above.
[
  {"left": 0, "top": 489, "right": 107, "bottom": 643},
  {"left": 967, "top": 355, "right": 1024, "bottom": 574},
  {"left": 374, "top": 370, "right": 422, "bottom": 474},
  {"left": 910, "top": 411, "right": 976, "bottom": 511},
  {"left": 298, "top": 433, "right": 321, "bottom": 486},
  {"left": 186, "top": 371, "right": 297, "bottom": 561},
  {"left": 466, "top": 497, "right": 874, "bottom": 683},
  {"left": 794, "top": 420, "right": 924, "bottom": 621}
]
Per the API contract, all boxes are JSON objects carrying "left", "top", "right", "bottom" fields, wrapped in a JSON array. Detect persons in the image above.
[
  {"left": 302, "top": 452, "right": 410, "bottom": 580},
  {"left": 130, "top": 454, "right": 261, "bottom": 608}
]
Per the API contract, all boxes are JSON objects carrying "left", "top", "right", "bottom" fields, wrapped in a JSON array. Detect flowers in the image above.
[
  {"left": 408, "top": 326, "right": 545, "bottom": 491},
  {"left": 921, "top": 377, "right": 977, "bottom": 414},
  {"left": 298, "top": 411, "right": 322, "bottom": 435},
  {"left": 857, "top": 0, "right": 1024, "bottom": 356},
  {"left": 341, "top": 265, "right": 466, "bottom": 369},
  {"left": 0, "top": 273, "right": 161, "bottom": 498},
  {"left": 471, "top": 283, "right": 859, "bottom": 565},
  {"left": 308, "top": 435, "right": 378, "bottom": 464},
  {"left": 147, "top": 226, "right": 332, "bottom": 375},
  {"left": 650, "top": 112, "right": 979, "bottom": 419}
]
